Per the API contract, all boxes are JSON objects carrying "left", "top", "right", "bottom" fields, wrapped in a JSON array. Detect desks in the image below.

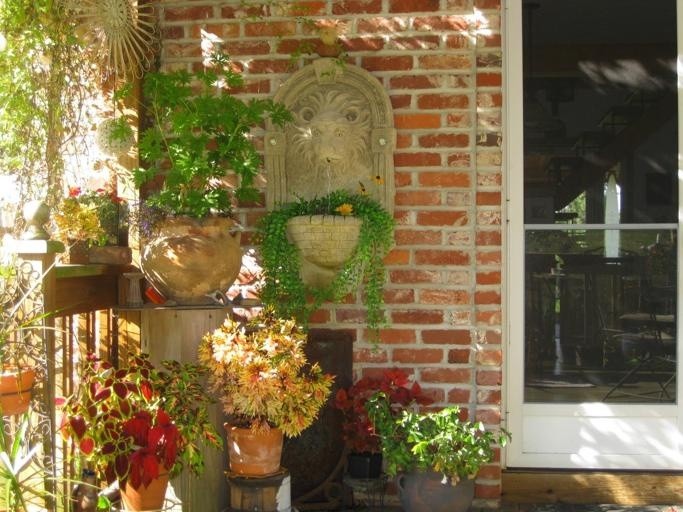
[{"left": 526, "top": 252, "right": 623, "bottom": 387}]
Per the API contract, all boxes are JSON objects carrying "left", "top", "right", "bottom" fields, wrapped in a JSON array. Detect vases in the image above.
[
  {"left": 346, "top": 451, "right": 381, "bottom": 477},
  {"left": 71, "top": 468, "right": 98, "bottom": 512},
  {"left": 60, "top": 240, "right": 88, "bottom": 266}
]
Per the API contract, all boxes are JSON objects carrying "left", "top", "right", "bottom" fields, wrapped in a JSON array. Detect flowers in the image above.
[{"left": 45, "top": 187, "right": 107, "bottom": 239}]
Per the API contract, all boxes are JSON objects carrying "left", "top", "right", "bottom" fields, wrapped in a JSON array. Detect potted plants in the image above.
[
  {"left": 252, "top": 190, "right": 395, "bottom": 359},
  {"left": 103, "top": 48, "right": 291, "bottom": 304},
  {"left": 56, "top": 353, "right": 223, "bottom": 512},
  {"left": 364, "top": 391, "right": 513, "bottom": 512},
  {"left": 196, "top": 305, "right": 336, "bottom": 475},
  {"left": 334, "top": 368, "right": 433, "bottom": 479},
  {"left": 0, "top": 240, "right": 79, "bottom": 415}
]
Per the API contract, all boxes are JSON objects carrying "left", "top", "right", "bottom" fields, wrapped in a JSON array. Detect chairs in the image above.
[
  {"left": 583, "top": 246, "right": 675, "bottom": 403},
  {"left": 617, "top": 249, "right": 676, "bottom": 331}
]
